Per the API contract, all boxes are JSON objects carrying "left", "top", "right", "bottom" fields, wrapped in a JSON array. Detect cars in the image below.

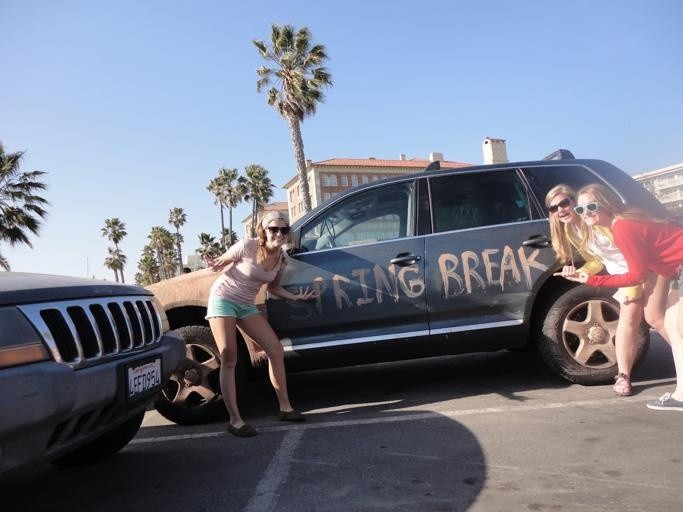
[
  {"left": 143, "top": 149, "right": 674, "bottom": 426},
  {"left": 0, "top": 270, "right": 187, "bottom": 489}
]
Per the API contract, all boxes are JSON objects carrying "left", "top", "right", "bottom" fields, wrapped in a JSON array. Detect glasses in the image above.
[
  {"left": 268, "top": 226, "right": 291, "bottom": 234},
  {"left": 574, "top": 202, "right": 601, "bottom": 216},
  {"left": 546, "top": 198, "right": 572, "bottom": 213}
]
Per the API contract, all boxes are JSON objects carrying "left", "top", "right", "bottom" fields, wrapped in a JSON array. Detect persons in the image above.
[
  {"left": 564, "top": 183, "right": 683, "bottom": 412},
  {"left": 545, "top": 184, "right": 672, "bottom": 396},
  {"left": 204, "top": 212, "right": 320, "bottom": 437}
]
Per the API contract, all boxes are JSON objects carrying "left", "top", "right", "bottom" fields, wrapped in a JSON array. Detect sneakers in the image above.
[
  {"left": 646, "top": 393, "right": 683, "bottom": 411},
  {"left": 277, "top": 410, "right": 306, "bottom": 421},
  {"left": 228, "top": 423, "right": 258, "bottom": 436},
  {"left": 612, "top": 373, "right": 633, "bottom": 396}
]
